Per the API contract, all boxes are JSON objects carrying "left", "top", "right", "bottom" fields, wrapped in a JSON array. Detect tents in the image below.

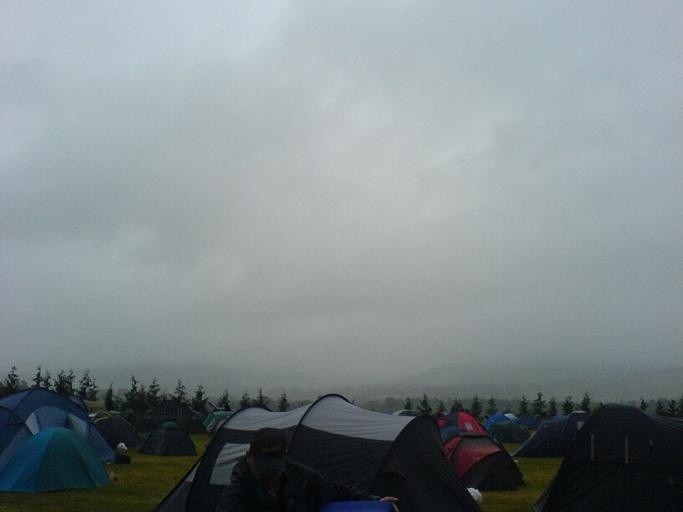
[{"left": 27, "top": 384, "right": 682, "bottom": 510}]
